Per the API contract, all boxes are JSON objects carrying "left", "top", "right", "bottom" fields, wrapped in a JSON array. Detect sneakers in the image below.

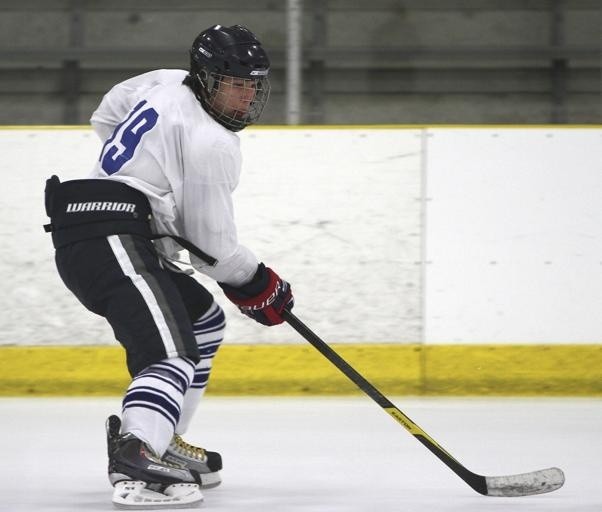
[{"left": 107, "top": 435, "right": 222, "bottom": 483}]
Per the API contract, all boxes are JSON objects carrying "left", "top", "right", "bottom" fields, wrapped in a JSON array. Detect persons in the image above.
[{"left": 41, "top": 26, "right": 294, "bottom": 486}]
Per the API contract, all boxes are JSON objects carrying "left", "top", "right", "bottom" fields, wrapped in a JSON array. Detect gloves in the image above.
[{"left": 216, "top": 263, "right": 293, "bottom": 326}]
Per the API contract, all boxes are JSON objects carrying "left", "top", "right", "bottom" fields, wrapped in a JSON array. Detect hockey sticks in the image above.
[{"left": 281, "top": 310, "right": 565, "bottom": 496}]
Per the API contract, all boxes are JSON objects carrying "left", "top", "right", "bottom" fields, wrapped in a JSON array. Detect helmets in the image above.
[{"left": 189, "top": 25, "right": 271, "bottom": 131}]
